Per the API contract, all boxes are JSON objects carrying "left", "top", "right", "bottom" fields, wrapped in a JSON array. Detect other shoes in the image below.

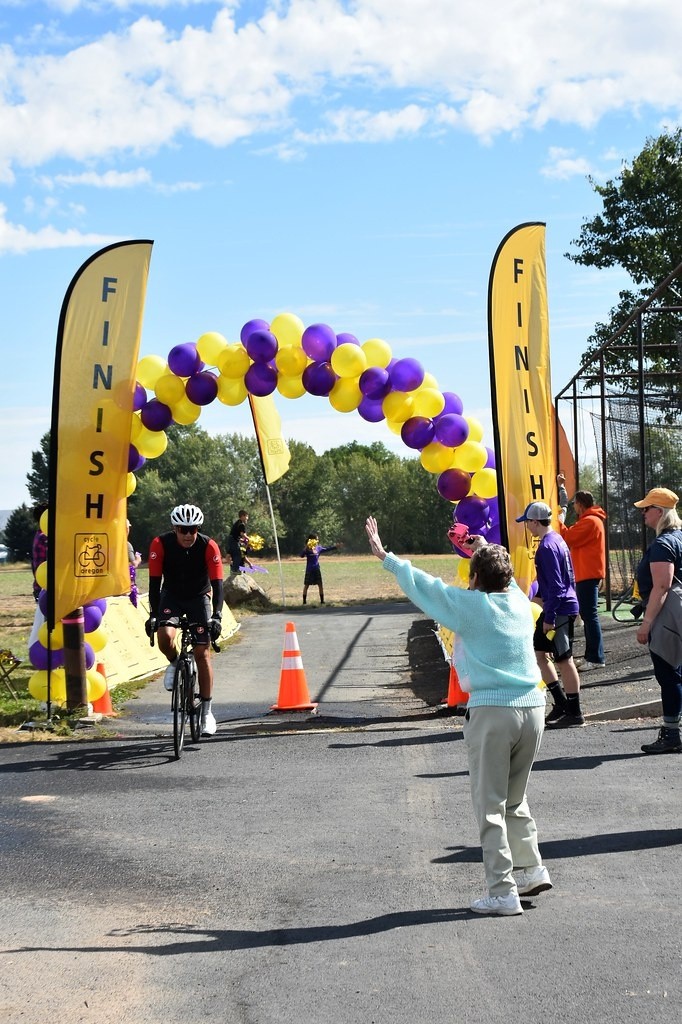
[{"left": 230, "top": 570, "right": 242, "bottom": 575}]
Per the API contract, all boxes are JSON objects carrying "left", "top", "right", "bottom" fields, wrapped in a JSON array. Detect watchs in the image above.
[{"left": 211, "top": 612, "right": 223, "bottom": 620}]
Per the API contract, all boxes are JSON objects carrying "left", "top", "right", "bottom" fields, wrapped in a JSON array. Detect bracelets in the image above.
[{"left": 643, "top": 618, "right": 653, "bottom": 625}]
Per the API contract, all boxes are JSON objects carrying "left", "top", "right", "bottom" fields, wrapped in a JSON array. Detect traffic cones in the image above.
[
  {"left": 437, "top": 657, "right": 470, "bottom": 717},
  {"left": 267, "top": 620, "right": 319, "bottom": 711},
  {"left": 90, "top": 663, "right": 117, "bottom": 717}
]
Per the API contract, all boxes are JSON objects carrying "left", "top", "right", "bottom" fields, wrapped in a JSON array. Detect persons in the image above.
[
  {"left": 32, "top": 524, "right": 48, "bottom": 603},
  {"left": 515, "top": 501, "right": 586, "bottom": 728},
  {"left": 300, "top": 534, "right": 343, "bottom": 604},
  {"left": 365, "top": 517, "right": 554, "bottom": 916},
  {"left": 145, "top": 504, "right": 224, "bottom": 736},
  {"left": 550, "top": 473, "right": 606, "bottom": 671},
  {"left": 226, "top": 548, "right": 253, "bottom": 575},
  {"left": 127, "top": 519, "right": 141, "bottom": 569},
  {"left": 224, "top": 510, "right": 254, "bottom": 576},
  {"left": 634, "top": 488, "right": 682, "bottom": 753}
]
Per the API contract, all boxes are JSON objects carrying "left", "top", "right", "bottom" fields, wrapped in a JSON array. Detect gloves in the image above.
[
  {"left": 210, "top": 613, "right": 222, "bottom": 640},
  {"left": 145, "top": 612, "right": 160, "bottom": 637}
]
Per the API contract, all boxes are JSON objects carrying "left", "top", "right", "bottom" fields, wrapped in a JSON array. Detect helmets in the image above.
[{"left": 171, "top": 504, "right": 204, "bottom": 526}]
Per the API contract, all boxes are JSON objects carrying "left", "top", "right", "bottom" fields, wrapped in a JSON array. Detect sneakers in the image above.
[
  {"left": 575, "top": 657, "right": 587, "bottom": 666},
  {"left": 201, "top": 713, "right": 216, "bottom": 735},
  {"left": 545, "top": 703, "right": 566, "bottom": 725},
  {"left": 547, "top": 710, "right": 586, "bottom": 727},
  {"left": 578, "top": 661, "right": 605, "bottom": 671},
  {"left": 470, "top": 891, "right": 524, "bottom": 915},
  {"left": 164, "top": 662, "right": 182, "bottom": 691},
  {"left": 517, "top": 866, "right": 553, "bottom": 897},
  {"left": 641, "top": 727, "right": 682, "bottom": 755}
]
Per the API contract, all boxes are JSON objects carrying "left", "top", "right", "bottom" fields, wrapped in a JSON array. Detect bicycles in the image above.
[{"left": 148, "top": 608, "right": 222, "bottom": 760}]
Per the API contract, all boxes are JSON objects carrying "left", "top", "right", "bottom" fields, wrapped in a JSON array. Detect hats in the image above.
[
  {"left": 634, "top": 488, "right": 679, "bottom": 508},
  {"left": 127, "top": 518, "right": 131, "bottom": 527},
  {"left": 515, "top": 501, "right": 552, "bottom": 523}
]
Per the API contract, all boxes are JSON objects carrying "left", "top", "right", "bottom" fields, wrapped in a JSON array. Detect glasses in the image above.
[
  {"left": 175, "top": 525, "right": 198, "bottom": 535},
  {"left": 571, "top": 501, "right": 576, "bottom": 505},
  {"left": 644, "top": 506, "right": 658, "bottom": 513}
]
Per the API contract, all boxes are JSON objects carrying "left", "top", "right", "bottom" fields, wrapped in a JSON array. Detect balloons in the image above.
[{"left": 28, "top": 313, "right": 506, "bottom": 704}]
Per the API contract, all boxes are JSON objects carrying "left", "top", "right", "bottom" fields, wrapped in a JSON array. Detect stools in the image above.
[{"left": 0, "top": 658, "right": 24, "bottom": 701}]
